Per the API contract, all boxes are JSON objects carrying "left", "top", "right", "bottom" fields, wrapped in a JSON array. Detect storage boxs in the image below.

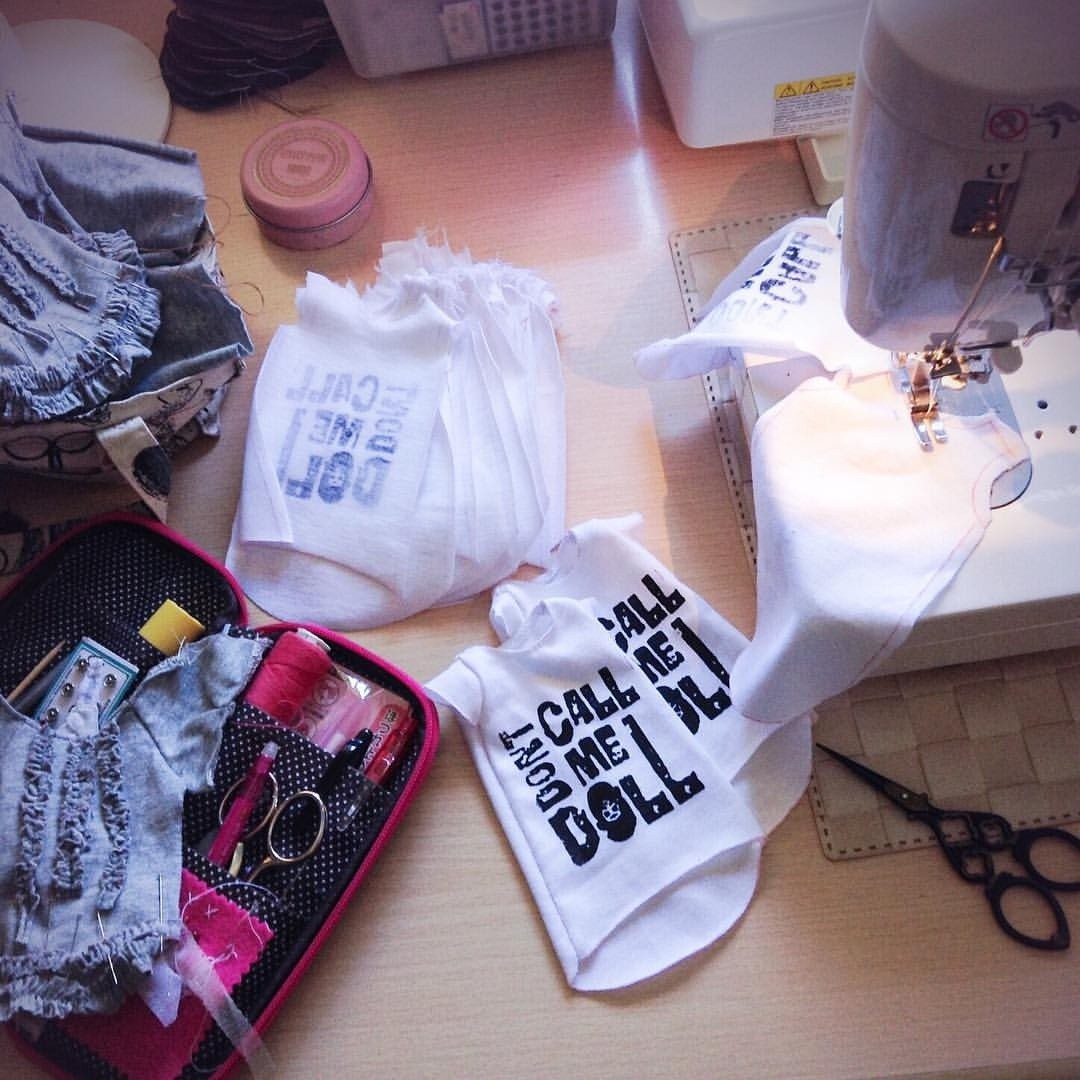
[{"left": 1, "top": 516, "right": 442, "bottom": 1080}]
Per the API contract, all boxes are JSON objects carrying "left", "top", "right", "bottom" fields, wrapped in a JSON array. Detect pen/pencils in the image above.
[
  {"left": 291, "top": 728, "right": 372, "bottom": 838},
  {"left": 211, "top": 742, "right": 278, "bottom": 868},
  {"left": 334, "top": 714, "right": 418, "bottom": 831}
]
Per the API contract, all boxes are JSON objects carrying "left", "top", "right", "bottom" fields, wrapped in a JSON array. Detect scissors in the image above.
[
  {"left": 219, "top": 769, "right": 327, "bottom": 885},
  {"left": 815, "top": 739, "right": 1080, "bottom": 950}
]
